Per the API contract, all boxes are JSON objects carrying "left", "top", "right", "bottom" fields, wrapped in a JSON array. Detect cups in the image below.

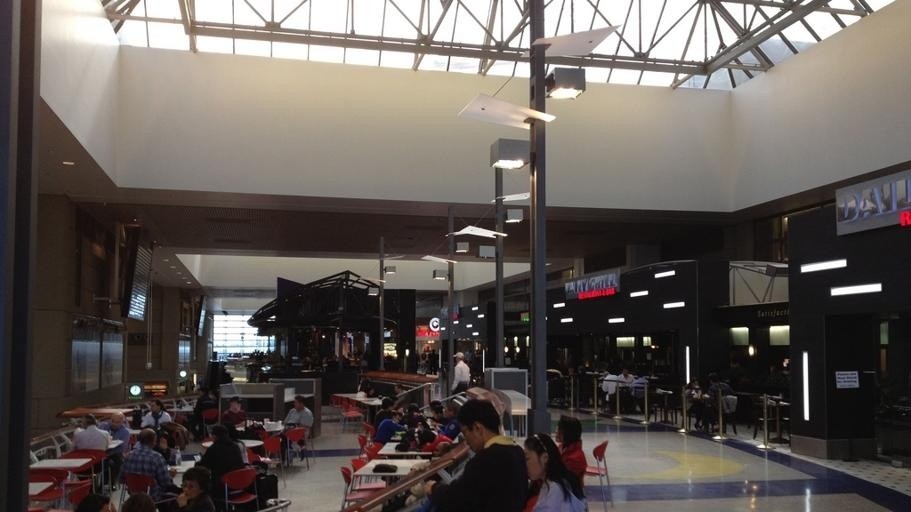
[{"left": 264, "top": 418, "right": 270, "bottom": 427}]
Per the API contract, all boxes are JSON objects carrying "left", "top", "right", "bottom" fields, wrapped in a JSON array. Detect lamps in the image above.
[
  {"left": 433, "top": 270, "right": 450, "bottom": 281},
  {"left": 489, "top": 138, "right": 530, "bottom": 169},
  {"left": 455, "top": 240, "right": 469, "bottom": 253},
  {"left": 505, "top": 208, "right": 524, "bottom": 223},
  {"left": 546, "top": 66, "right": 587, "bottom": 100},
  {"left": 383, "top": 265, "right": 396, "bottom": 275},
  {"left": 368, "top": 287, "right": 380, "bottom": 297}
]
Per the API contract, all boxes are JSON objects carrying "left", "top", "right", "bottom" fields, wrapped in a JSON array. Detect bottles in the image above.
[
  {"left": 366, "top": 430, "right": 374, "bottom": 449},
  {"left": 175, "top": 447, "right": 182, "bottom": 467}
]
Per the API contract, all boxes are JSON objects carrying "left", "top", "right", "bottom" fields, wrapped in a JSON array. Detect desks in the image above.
[
  {"left": 353, "top": 420, "right": 438, "bottom": 487},
  {"left": 219, "top": 382, "right": 285, "bottom": 422},
  {"left": 227, "top": 355, "right": 252, "bottom": 369},
  {"left": 269, "top": 377, "right": 321, "bottom": 438}
]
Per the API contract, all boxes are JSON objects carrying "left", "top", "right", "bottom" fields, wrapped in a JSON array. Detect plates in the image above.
[{"left": 265, "top": 422, "right": 282, "bottom": 430}]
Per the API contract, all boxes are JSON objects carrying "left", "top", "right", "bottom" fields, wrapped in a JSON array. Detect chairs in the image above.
[
  {"left": 546, "top": 367, "right": 791, "bottom": 444},
  {"left": 27, "top": 407, "right": 315, "bottom": 511},
  {"left": 586, "top": 440, "right": 614, "bottom": 512},
  {"left": 330, "top": 394, "right": 389, "bottom": 512}
]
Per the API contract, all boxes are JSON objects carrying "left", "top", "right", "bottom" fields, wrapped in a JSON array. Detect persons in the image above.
[{"left": 67, "top": 350, "right": 739, "bottom": 510}]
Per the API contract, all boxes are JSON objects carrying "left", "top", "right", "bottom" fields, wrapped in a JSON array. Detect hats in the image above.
[{"left": 453, "top": 352, "right": 464, "bottom": 360}]
